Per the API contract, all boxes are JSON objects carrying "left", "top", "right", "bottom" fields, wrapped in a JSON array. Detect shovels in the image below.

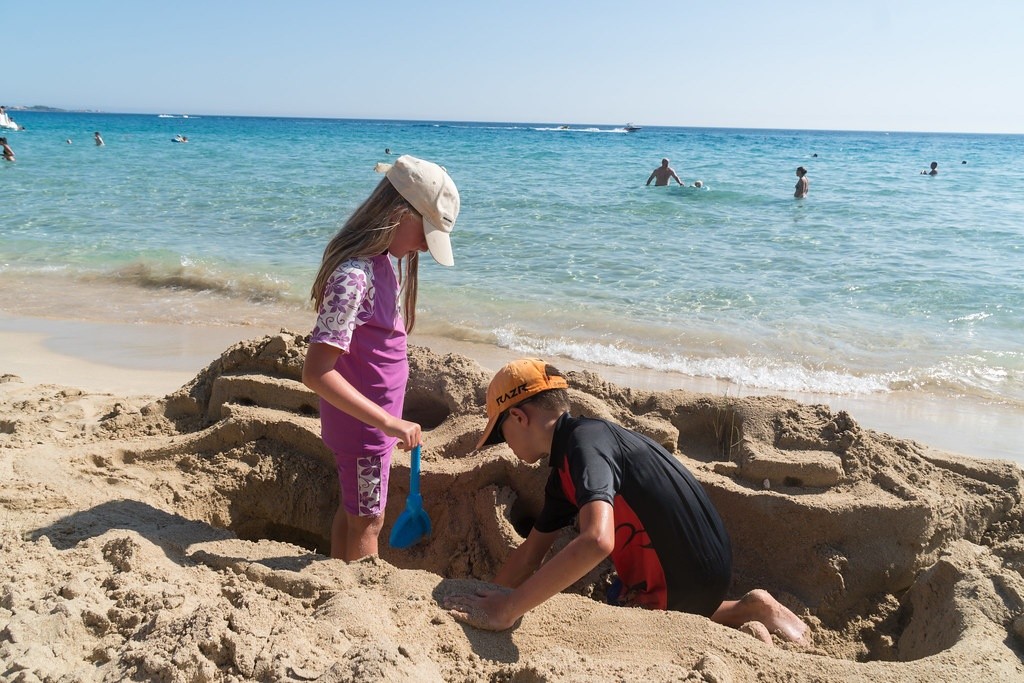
[{"left": 387, "top": 443, "right": 432, "bottom": 551}]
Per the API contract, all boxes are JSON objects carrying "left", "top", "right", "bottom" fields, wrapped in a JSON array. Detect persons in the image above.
[
  {"left": 928, "top": 162, "right": 939, "bottom": 176},
  {"left": 94, "top": 132, "right": 105, "bottom": 146},
  {"left": 301, "top": 151, "right": 462, "bottom": 564},
  {"left": 385, "top": 148, "right": 392, "bottom": 154},
  {"left": 175, "top": 134, "right": 189, "bottom": 142},
  {"left": 813, "top": 153, "right": 818, "bottom": 157},
  {"left": 0, "top": 137, "right": 15, "bottom": 161},
  {"left": 646, "top": 158, "right": 686, "bottom": 189},
  {"left": 794, "top": 167, "right": 809, "bottom": 198},
  {"left": 962, "top": 161, "right": 967, "bottom": 164},
  {"left": 445, "top": 357, "right": 812, "bottom": 651},
  {"left": 694, "top": 180, "right": 702, "bottom": 188},
  {"left": 0, "top": 105, "right": 26, "bottom": 132},
  {"left": 67, "top": 139, "right": 73, "bottom": 144}
]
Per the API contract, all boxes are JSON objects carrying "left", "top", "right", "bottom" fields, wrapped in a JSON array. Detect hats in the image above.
[
  {"left": 385, "top": 154, "right": 460, "bottom": 267},
  {"left": 475, "top": 357, "right": 570, "bottom": 449}
]
